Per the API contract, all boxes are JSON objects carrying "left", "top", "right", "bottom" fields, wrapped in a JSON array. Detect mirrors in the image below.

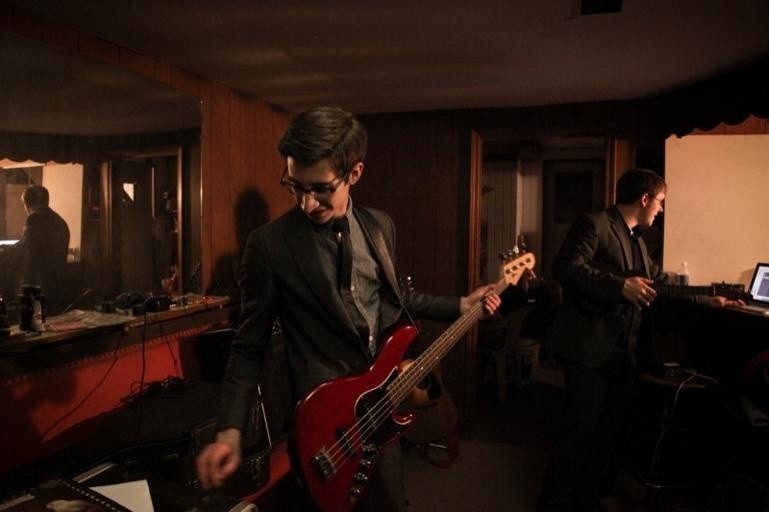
[{"left": 1, "top": 134, "right": 192, "bottom": 320}]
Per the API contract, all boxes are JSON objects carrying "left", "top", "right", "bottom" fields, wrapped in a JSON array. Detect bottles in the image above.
[
  {"left": 16, "top": 285, "right": 28, "bottom": 331},
  {"left": 27, "top": 286, "right": 48, "bottom": 331},
  {"left": 680, "top": 261, "right": 689, "bottom": 286}
]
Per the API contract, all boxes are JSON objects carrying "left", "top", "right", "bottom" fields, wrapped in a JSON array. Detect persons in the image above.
[
  {"left": 537, "top": 165, "right": 746, "bottom": 512},
  {"left": 198, "top": 106, "right": 503, "bottom": 512},
  {"left": 0, "top": 184, "right": 71, "bottom": 314}
]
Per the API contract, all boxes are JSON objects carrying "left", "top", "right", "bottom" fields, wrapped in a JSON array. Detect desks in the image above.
[{"left": 0, "top": 292, "right": 231, "bottom": 500}]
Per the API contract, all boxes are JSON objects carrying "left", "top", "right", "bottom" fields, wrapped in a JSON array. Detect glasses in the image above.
[
  {"left": 654, "top": 198, "right": 664, "bottom": 206},
  {"left": 281, "top": 165, "right": 348, "bottom": 200}
]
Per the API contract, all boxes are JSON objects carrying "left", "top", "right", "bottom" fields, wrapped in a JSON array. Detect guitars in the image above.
[
  {"left": 571, "top": 261, "right": 745, "bottom": 313},
  {"left": 396, "top": 272, "right": 461, "bottom": 445},
  {"left": 288, "top": 244, "right": 537, "bottom": 499}
]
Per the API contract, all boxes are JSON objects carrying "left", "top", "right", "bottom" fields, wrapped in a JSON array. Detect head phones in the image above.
[
  {"left": 145, "top": 293, "right": 170, "bottom": 311},
  {"left": 116, "top": 290, "right": 143, "bottom": 309}
]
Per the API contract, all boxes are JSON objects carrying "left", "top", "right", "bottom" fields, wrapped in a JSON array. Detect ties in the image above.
[
  {"left": 332, "top": 217, "right": 369, "bottom": 350},
  {"left": 630, "top": 236, "right": 642, "bottom": 274}
]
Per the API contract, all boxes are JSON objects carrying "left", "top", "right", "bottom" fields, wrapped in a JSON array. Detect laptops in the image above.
[
  {"left": 0, "top": 237, "right": 20, "bottom": 248},
  {"left": 726, "top": 261, "right": 769, "bottom": 313}
]
